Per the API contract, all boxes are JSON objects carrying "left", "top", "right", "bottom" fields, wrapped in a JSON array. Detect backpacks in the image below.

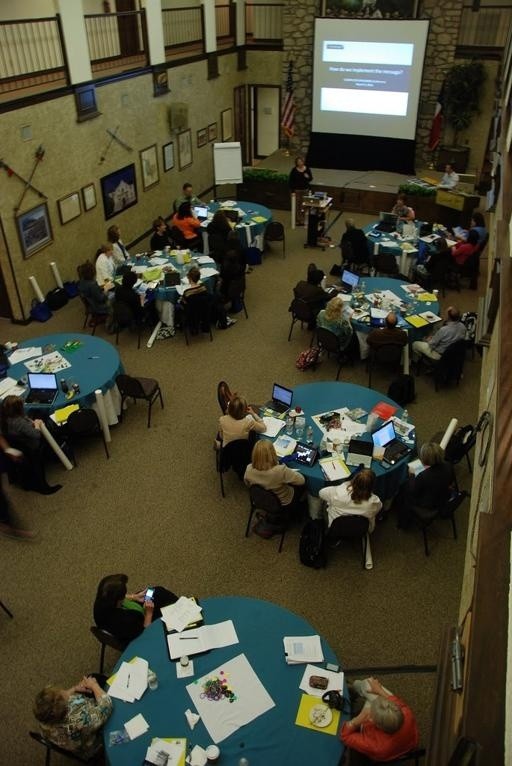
[
  {"left": 296, "top": 347, "right": 321, "bottom": 371},
  {"left": 299, "top": 518, "right": 327, "bottom": 570},
  {"left": 432, "top": 356, "right": 462, "bottom": 390}
]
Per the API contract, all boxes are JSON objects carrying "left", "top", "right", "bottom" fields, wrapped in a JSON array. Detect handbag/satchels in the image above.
[
  {"left": 63, "top": 280, "right": 80, "bottom": 300},
  {"left": 29, "top": 299, "right": 53, "bottom": 323},
  {"left": 46, "top": 285, "right": 69, "bottom": 311}
]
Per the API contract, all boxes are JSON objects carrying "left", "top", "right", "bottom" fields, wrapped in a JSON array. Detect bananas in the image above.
[{"left": 67, "top": 390, "right": 73, "bottom": 400}]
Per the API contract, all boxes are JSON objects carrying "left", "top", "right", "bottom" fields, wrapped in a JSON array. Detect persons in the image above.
[
  {"left": 404, "top": 443, "right": 459, "bottom": 515},
  {"left": 217, "top": 397, "right": 267, "bottom": 452},
  {"left": 341, "top": 677, "right": 420, "bottom": 762},
  {"left": 289, "top": 157, "right": 313, "bottom": 226},
  {"left": 340, "top": 218, "right": 368, "bottom": 265},
  {"left": 1, "top": 396, "right": 62, "bottom": 495},
  {"left": 413, "top": 306, "right": 467, "bottom": 364},
  {"left": 319, "top": 468, "right": 382, "bottom": 535},
  {"left": 391, "top": 193, "right": 415, "bottom": 223},
  {"left": 33, "top": 674, "right": 113, "bottom": 759},
  {"left": 365, "top": 313, "right": 408, "bottom": 372},
  {"left": 316, "top": 296, "right": 354, "bottom": 357},
  {"left": 294, "top": 266, "right": 344, "bottom": 331},
  {"left": 244, "top": 438, "right": 306, "bottom": 519},
  {"left": 79, "top": 182, "right": 252, "bottom": 337},
  {"left": 93, "top": 573, "right": 179, "bottom": 643},
  {"left": 438, "top": 164, "right": 459, "bottom": 189},
  {"left": 422, "top": 212, "right": 488, "bottom": 286}
]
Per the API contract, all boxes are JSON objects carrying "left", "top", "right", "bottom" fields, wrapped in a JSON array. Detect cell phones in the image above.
[{"left": 144, "top": 587, "right": 156, "bottom": 600}]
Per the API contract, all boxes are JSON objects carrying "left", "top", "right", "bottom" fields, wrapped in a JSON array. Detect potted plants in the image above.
[{"left": 437, "top": 64, "right": 475, "bottom": 173}]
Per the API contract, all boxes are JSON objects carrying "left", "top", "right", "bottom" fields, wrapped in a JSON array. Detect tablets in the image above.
[{"left": 292, "top": 440, "right": 321, "bottom": 466}]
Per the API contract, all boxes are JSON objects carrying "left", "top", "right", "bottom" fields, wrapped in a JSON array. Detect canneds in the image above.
[
  {"left": 373, "top": 298, "right": 382, "bottom": 309},
  {"left": 71, "top": 383, "right": 80, "bottom": 395}
]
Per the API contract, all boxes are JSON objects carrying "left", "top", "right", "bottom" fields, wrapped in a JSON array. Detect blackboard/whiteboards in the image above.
[{"left": 212, "top": 140, "right": 244, "bottom": 186}]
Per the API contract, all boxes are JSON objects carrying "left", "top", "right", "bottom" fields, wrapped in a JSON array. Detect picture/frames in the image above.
[
  {"left": 58, "top": 191, "right": 81, "bottom": 224},
  {"left": 16, "top": 202, "right": 54, "bottom": 260},
  {"left": 163, "top": 141, "right": 174, "bottom": 172},
  {"left": 208, "top": 122, "right": 217, "bottom": 142},
  {"left": 178, "top": 129, "right": 193, "bottom": 171},
  {"left": 221, "top": 108, "right": 233, "bottom": 142},
  {"left": 82, "top": 183, "right": 96, "bottom": 212},
  {"left": 100, "top": 163, "right": 138, "bottom": 220},
  {"left": 139, "top": 143, "right": 160, "bottom": 192},
  {"left": 196, "top": 128, "right": 207, "bottom": 148}
]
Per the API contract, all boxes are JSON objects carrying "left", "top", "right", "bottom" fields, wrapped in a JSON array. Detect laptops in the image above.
[
  {"left": 333, "top": 269, "right": 358, "bottom": 294},
  {"left": 265, "top": 382, "right": 294, "bottom": 415},
  {"left": 371, "top": 420, "right": 413, "bottom": 465},
  {"left": 194, "top": 206, "right": 208, "bottom": 222},
  {"left": 24, "top": 372, "right": 58, "bottom": 405}
]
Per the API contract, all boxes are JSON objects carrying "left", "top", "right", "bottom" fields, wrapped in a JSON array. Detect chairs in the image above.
[
  {"left": 116, "top": 375, "right": 163, "bottom": 427},
  {"left": 478, "top": 232, "right": 489, "bottom": 257},
  {"left": 31, "top": 731, "right": 89, "bottom": 766},
  {"left": 218, "top": 261, "right": 248, "bottom": 319},
  {"left": 322, "top": 515, "right": 369, "bottom": 569},
  {"left": 288, "top": 299, "right": 316, "bottom": 348},
  {"left": 215, "top": 440, "right": 252, "bottom": 499},
  {"left": 313, "top": 328, "right": 352, "bottom": 381},
  {"left": 425, "top": 349, "right": 462, "bottom": 391},
  {"left": 374, "top": 254, "right": 400, "bottom": 279},
  {"left": 91, "top": 627, "right": 130, "bottom": 673},
  {"left": 349, "top": 243, "right": 370, "bottom": 272},
  {"left": 112, "top": 302, "right": 153, "bottom": 349},
  {"left": 264, "top": 222, "right": 286, "bottom": 258},
  {"left": 406, "top": 490, "right": 467, "bottom": 556},
  {"left": 436, "top": 263, "right": 461, "bottom": 296},
  {"left": 178, "top": 293, "right": 214, "bottom": 345},
  {"left": 246, "top": 485, "right": 295, "bottom": 553},
  {"left": 173, "top": 200, "right": 176, "bottom": 212},
  {"left": 82, "top": 297, "right": 107, "bottom": 336},
  {"left": 368, "top": 343, "right": 402, "bottom": 389},
  {"left": 171, "top": 227, "right": 200, "bottom": 251},
  {"left": 459, "top": 251, "right": 480, "bottom": 289},
  {"left": 61, "top": 408, "right": 109, "bottom": 466},
  {"left": 461, "top": 312, "right": 478, "bottom": 357},
  {"left": 429, "top": 431, "right": 477, "bottom": 490},
  {"left": 218, "top": 382, "right": 230, "bottom": 415},
  {"left": 159, "top": 216, "right": 171, "bottom": 238}
]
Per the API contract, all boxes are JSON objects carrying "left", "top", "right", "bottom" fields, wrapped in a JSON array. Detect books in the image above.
[
  {"left": 319, "top": 455, "right": 351, "bottom": 482},
  {"left": 166, "top": 620, "right": 239, "bottom": 661},
  {"left": 404, "top": 310, "right": 444, "bottom": 329}
]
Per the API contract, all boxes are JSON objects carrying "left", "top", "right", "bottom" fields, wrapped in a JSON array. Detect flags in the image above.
[
  {"left": 280, "top": 68, "right": 298, "bottom": 138},
  {"left": 428, "top": 80, "right": 446, "bottom": 152}
]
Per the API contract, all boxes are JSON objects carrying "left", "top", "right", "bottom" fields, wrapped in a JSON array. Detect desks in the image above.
[
  {"left": 343, "top": 277, "right": 440, "bottom": 357},
  {"left": 113, "top": 249, "right": 217, "bottom": 327},
  {"left": 259, "top": 381, "right": 416, "bottom": 503},
  {"left": 103, "top": 595, "right": 350, "bottom": 766},
  {"left": 0, "top": 334, "right": 126, "bottom": 424},
  {"left": 304, "top": 197, "right": 332, "bottom": 250},
  {"left": 194, "top": 202, "right": 271, "bottom": 252},
  {"left": 363, "top": 220, "right": 436, "bottom": 266}
]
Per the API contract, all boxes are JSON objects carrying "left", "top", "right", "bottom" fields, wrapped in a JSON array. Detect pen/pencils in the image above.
[
  {"left": 179, "top": 637, "right": 198, "bottom": 639},
  {"left": 0, "top": 388, "right": 10, "bottom": 397},
  {"left": 88, "top": 357, "right": 100, "bottom": 359},
  {"left": 127, "top": 673, "right": 130, "bottom": 688},
  {"left": 332, "top": 461, "right": 336, "bottom": 470}
]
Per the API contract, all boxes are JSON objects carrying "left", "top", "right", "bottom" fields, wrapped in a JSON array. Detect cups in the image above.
[
  {"left": 163, "top": 249, "right": 170, "bottom": 258},
  {"left": 147, "top": 673, "right": 158, "bottom": 690},
  {"left": 295, "top": 422, "right": 305, "bottom": 440},
  {"left": 5, "top": 342, "right": 12, "bottom": 350}
]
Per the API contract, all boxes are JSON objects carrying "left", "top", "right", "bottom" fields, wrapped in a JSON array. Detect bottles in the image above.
[
  {"left": 306, "top": 425, "right": 314, "bottom": 445},
  {"left": 286, "top": 418, "right": 294, "bottom": 435},
  {"left": 402, "top": 409, "right": 409, "bottom": 424},
  {"left": 176, "top": 245, "right": 185, "bottom": 266},
  {"left": 344, "top": 436, "right": 350, "bottom": 456},
  {"left": 181, "top": 654, "right": 190, "bottom": 673},
  {"left": 60, "top": 378, "right": 68, "bottom": 393}
]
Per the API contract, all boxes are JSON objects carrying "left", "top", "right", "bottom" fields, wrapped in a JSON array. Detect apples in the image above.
[{"left": 295, "top": 406, "right": 301, "bottom": 413}]
